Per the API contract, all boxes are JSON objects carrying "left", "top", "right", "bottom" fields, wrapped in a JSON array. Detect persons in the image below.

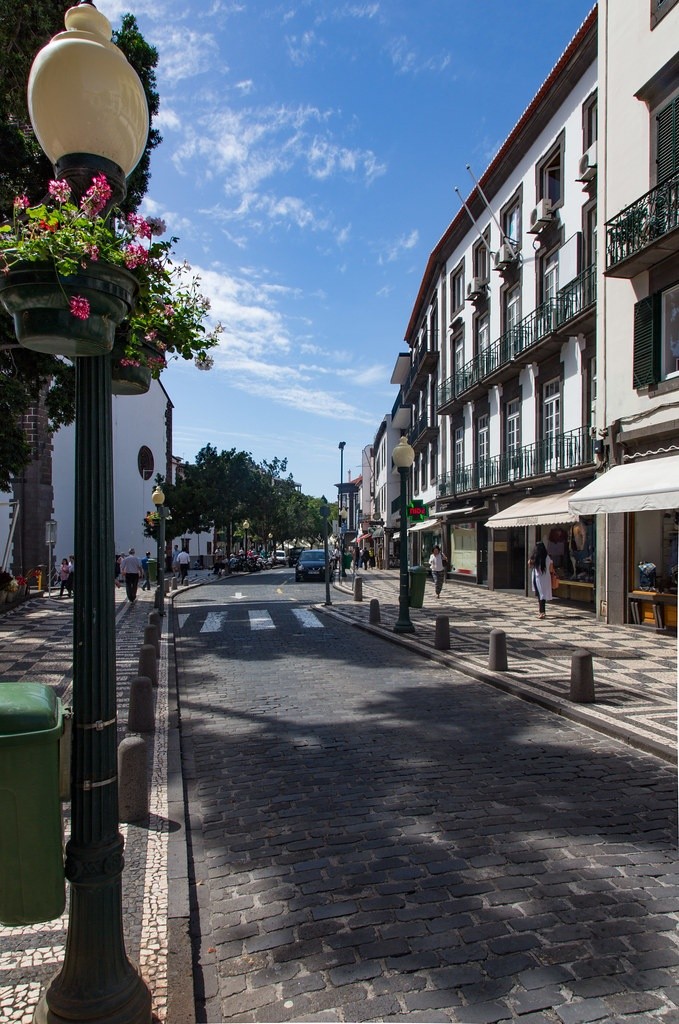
[
  {"left": 115, "top": 547, "right": 151, "bottom": 606},
  {"left": 171, "top": 545, "right": 191, "bottom": 584},
  {"left": 332, "top": 546, "right": 374, "bottom": 570},
  {"left": 212, "top": 546, "right": 253, "bottom": 574},
  {"left": 528, "top": 542, "right": 554, "bottom": 619},
  {"left": 429, "top": 545, "right": 447, "bottom": 598},
  {"left": 58, "top": 555, "right": 74, "bottom": 598}
]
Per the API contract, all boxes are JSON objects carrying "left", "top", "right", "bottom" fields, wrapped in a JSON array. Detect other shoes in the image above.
[{"left": 538, "top": 613, "right": 545, "bottom": 619}]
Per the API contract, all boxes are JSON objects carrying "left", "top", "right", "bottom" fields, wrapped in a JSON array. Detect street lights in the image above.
[
  {"left": 27, "top": 2, "right": 159, "bottom": 1024},
  {"left": 391, "top": 437, "right": 419, "bottom": 632},
  {"left": 243, "top": 519, "right": 253, "bottom": 562},
  {"left": 151, "top": 486, "right": 167, "bottom": 597},
  {"left": 340, "top": 506, "right": 348, "bottom": 577},
  {"left": 338, "top": 441, "right": 346, "bottom": 511},
  {"left": 269, "top": 533, "right": 273, "bottom": 549}
]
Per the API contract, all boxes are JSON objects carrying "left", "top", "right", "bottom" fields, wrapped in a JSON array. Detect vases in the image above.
[
  {"left": 0, "top": 255, "right": 137, "bottom": 357},
  {"left": 112, "top": 320, "right": 168, "bottom": 397},
  {"left": 153, "top": 524, "right": 159, "bottom": 541}
]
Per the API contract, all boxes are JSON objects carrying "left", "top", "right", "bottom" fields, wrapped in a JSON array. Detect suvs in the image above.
[{"left": 288, "top": 547, "right": 305, "bottom": 567}]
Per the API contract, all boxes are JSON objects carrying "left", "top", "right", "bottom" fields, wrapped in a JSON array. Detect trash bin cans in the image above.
[
  {"left": 0, "top": 681, "right": 64, "bottom": 928},
  {"left": 407, "top": 565, "right": 428, "bottom": 609},
  {"left": 343, "top": 552, "right": 352, "bottom": 569},
  {"left": 146, "top": 559, "right": 157, "bottom": 583}
]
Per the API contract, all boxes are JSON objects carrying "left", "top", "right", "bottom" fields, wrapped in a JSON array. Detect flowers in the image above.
[
  {"left": 143, "top": 511, "right": 159, "bottom": 537},
  {"left": 0, "top": 169, "right": 151, "bottom": 321},
  {"left": 125, "top": 213, "right": 223, "bottom": 372}
]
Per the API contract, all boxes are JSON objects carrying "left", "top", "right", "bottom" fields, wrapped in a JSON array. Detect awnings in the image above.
[
  {"left": 484, "top": 489, "right": 580, "bottom": 528},
  {"left": 568, "top": 454, "right": 679, "bottom": 515},
  {"left": 393, "top": 507, "right": 487, "bottom": 539},
  {"left": 350, "top": 528, "right": 384, "bottom": 542}
]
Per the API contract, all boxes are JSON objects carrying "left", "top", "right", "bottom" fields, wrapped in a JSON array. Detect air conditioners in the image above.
[
  {"left": 577, "top": 140, "right": 597, "bottom": 179},
  {"left": 529, "top": 197, "right": 551, "bottom": 232},
  {"left": 466, "top": 277, "right": 482, "bottom": 299},
  {"left": 491, "top": 244, "right": 513, "bottom": 270}
]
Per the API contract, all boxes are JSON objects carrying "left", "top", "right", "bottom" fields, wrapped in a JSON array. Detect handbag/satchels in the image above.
[
  {"left": 551, "top": 571, "right": 560, "bottom": 589},
  {"left": 441, "top": 553, "right": 447, "bottom": 567}
]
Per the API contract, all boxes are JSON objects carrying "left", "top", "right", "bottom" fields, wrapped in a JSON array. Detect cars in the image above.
[
  {"left": 270, "top": 550, "right": 287, "bottom": 566},
  {"left": 295, "top": 550, "right": 334, "bottom": 583}
]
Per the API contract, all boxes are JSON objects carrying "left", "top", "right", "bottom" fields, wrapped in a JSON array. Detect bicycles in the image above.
[{"left": 232, "top": 556, "right": 272, "bottom": 572}]
